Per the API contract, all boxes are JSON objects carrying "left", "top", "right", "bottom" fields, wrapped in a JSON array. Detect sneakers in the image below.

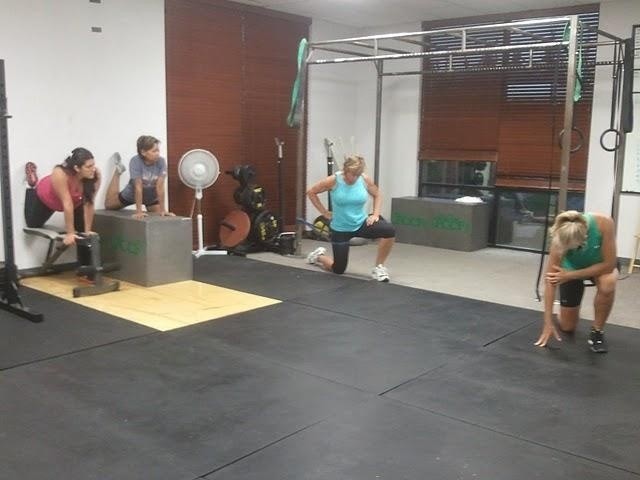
[
  {"left": 307, "top": 247, "right": 326, "bottom": 264},
  {"left": 372, "top": 264, "right": 390, "bottom": 282},
  {"left": 25, "top": 162, "right": 38, "bottom": 186},
  {"left": 112, "top": 152, "right": 126, "bottom": 174},
  {"left": 588, "top": 330, "right": 609, "bottom": 353}
]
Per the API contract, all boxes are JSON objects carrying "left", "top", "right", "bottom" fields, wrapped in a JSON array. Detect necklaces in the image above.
[{"left": 78, "top": 196, "right": 82, "bottom": 200}]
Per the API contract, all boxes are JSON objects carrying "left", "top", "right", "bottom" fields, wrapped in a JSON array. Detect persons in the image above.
[
  {"left": 24, "top": 147, "right": 101, "bottom": 248},
  {"left": 103, "top": 135, "right": 177, "bottom": 220},
  {"left": 532, "top": 207, "right": 619, "bottom": 355},
  {"left": 307, "top": 154, "right": 397, "bottom": 283}
]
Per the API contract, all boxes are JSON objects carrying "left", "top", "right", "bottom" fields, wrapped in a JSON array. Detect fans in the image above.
[{"left": 178, "top": 149, "right": 228, "bottom": 260}]
